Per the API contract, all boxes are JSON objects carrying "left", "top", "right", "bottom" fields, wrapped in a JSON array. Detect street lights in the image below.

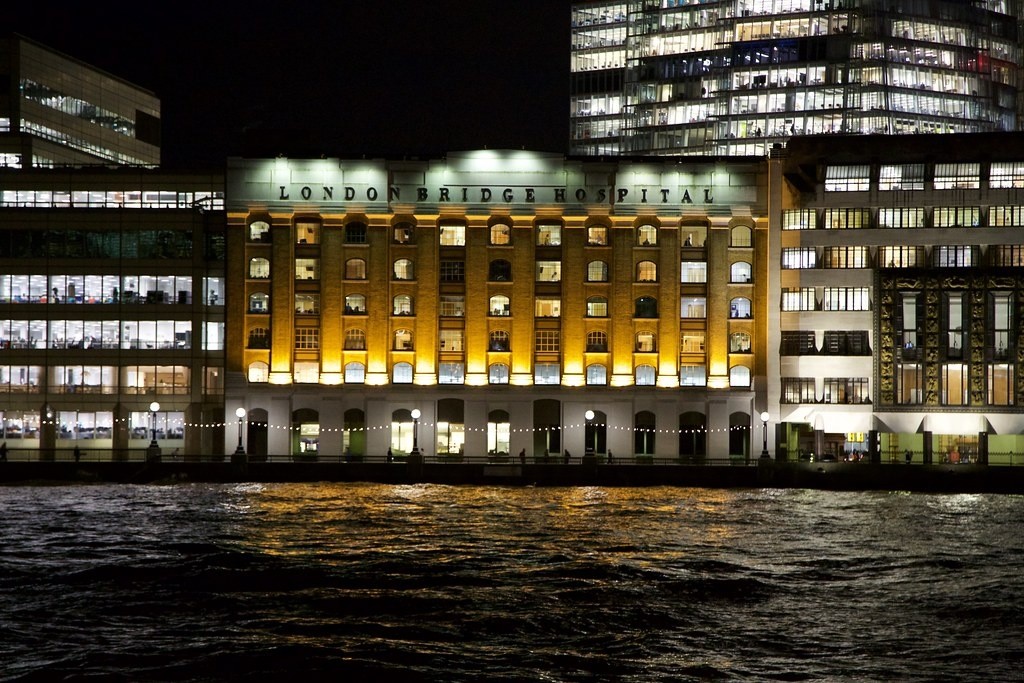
[
  {"left": 411, "top": 409, "right": 421, "bottom": 453},
  {"left": 585, "top": 410, "right": 595, "bottom": 455},
  {"left": 761, "top": 412, "right": 771, "bottom": 462},
  {"left": 235, "top": 407, "right": 246, "bottom": 446},
  {"left": 150, "top": 402, "right": 160, "bottom": 444}
]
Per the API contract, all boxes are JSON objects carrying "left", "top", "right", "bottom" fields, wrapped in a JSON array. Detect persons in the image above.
[
  {"left": 420, "top": 448, "right": 425, "bottom": 464},
  {"left": 607, "top": 449, "right": 613, "bottom": 464},
  {"left": 344, "top": 447, "right": 351, "bottom": 463},
  {"left": 520, "top": 448, "right": 526, "bottom": 464},
  {"left": 544, "top": 449, "right": 549, "bottom": 463},
  {"left": 564, "top": 449, "right": 570, "bottom": 464},
  {"left": 387, "top": 447, "right": 393, "bottom": 463}
]
